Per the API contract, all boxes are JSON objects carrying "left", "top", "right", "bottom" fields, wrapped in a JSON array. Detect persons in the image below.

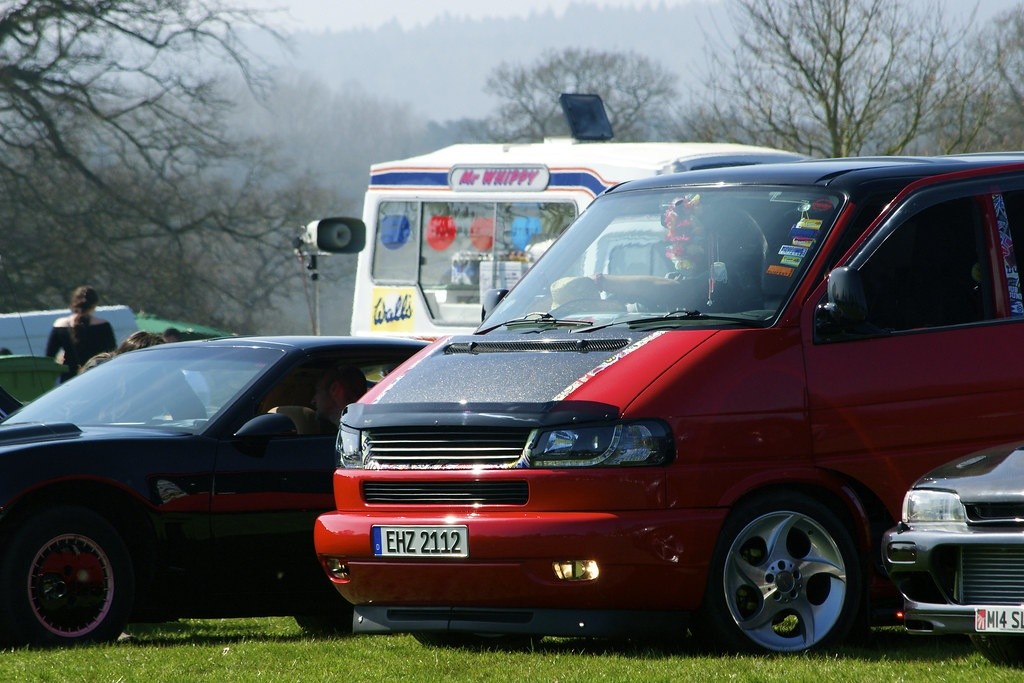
[
  {"left": 587, "top": 223, "right": 766, "bottom": 315},
  {"left": 45, "top": 285, "right": 181, "bottom": 423}
]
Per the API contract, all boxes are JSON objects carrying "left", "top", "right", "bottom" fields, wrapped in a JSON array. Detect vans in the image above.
[
  {"left": 348, "top": 92, "right": 838, "bottom": 378},
  {"left": 315, "top": 146, "right": 1024, "bottom": 660}
]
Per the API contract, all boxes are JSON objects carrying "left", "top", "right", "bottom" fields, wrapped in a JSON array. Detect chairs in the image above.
[{"left": 268, "top": 405, "right": 318, "bottom": 434}]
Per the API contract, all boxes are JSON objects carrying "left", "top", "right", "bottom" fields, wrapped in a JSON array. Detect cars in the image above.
[
  {"left": 881, "top": 438, "right": 1024, "bottom": 668},
  {"left": 0, "top": 305, "right": 241, "bottom": 424},
  {"left": 0, "top": 335, "right": 438, "bottom": 650}
]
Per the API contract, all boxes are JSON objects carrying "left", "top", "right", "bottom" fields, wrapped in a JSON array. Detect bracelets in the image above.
[{"left": 595, "top": 272, "right": 604, "bottom": 288}]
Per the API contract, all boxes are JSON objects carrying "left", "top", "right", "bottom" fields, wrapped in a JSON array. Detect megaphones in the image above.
[{"left": 303, "top": 217, "right": 367, "bottom": 256}]
